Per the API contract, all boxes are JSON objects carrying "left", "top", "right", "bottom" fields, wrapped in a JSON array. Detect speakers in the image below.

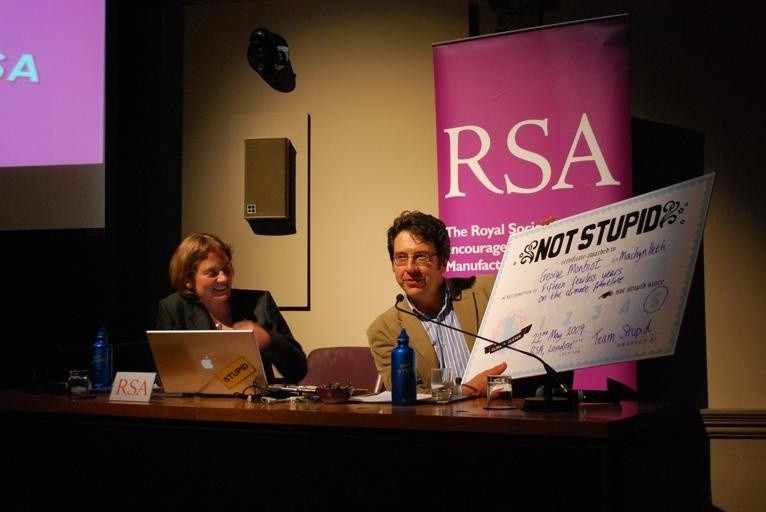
[{"left": 243, "top": 137, "right": 292, "bottom": 221}]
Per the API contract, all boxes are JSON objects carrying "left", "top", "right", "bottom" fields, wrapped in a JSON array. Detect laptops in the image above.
[{"left": 145, "top": 330, "right": 292, "bottom": 396}]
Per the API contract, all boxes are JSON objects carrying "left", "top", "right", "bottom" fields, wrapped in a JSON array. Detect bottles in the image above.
[
  {"left": 91, "top": 325, "right": 115, "bottom": 392},
  {"left": 390, "top": 329, "right": 416, "bottom": 406}
]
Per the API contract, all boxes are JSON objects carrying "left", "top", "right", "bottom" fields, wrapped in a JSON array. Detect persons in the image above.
[
  {"left": 155, "top": 232, "right": 306, "bottom": 384},
  {"left": 366, "top": 210, "right": 574, "bottom": 398}
]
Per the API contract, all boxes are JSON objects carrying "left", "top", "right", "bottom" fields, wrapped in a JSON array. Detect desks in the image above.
[{"left": 0, "top": 386, "right": 673, "bottom": 445}]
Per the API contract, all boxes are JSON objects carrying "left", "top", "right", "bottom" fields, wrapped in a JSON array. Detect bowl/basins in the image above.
[{"left": 318, "top": 384, "right": 352, "bottom": 404}]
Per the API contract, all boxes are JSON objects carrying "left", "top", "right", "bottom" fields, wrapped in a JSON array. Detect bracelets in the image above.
[{"left": 463, "top": 384, "right": 484, "bottom": 397}]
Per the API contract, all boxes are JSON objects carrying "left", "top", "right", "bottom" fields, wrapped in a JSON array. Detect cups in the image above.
[
  {"left": 484, "top": 374, "right": 512, "bottom": 409},
  {"left": 430, "top": 368, "right": 454, "bottom": 403},
  {"left": 67, "top": 368, "right": 89, "bottom": 397}
]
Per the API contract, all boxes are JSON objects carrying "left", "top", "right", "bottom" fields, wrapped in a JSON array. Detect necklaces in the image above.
[{"left": 208, "top": 311, "right": 233, "bottom": 323}]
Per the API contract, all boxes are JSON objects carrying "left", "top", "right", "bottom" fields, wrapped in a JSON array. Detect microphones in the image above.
[{"left": 393, "top": 293, "right": 579, "bottom": 413}]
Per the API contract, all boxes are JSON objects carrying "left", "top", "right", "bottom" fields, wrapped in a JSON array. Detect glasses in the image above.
[{"left": 392, "top": 252, "right": 439, "bottom": 266}]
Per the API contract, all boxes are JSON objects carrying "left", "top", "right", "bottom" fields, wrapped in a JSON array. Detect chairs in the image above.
[{"left": 295, "top": 345, "right": 384, "bottom": 395}]
